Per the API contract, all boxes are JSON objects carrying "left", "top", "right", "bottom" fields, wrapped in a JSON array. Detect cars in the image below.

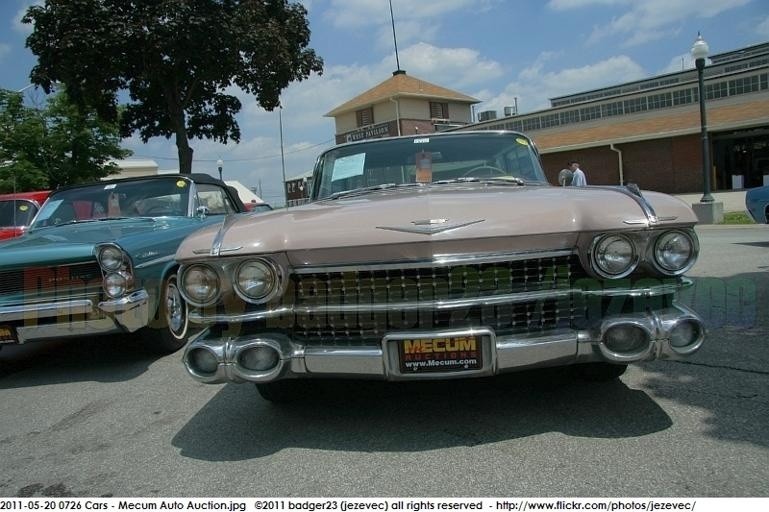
[
  {"left": 745, "top": 185, "right": 768, "bottom": 225},
  {"left": 0, "top": 191, "right": 141, "bottom": 242},
  {"left": 0, "top": 173, "right": 273, "bottom": 353},
  {"left": 175, "top": 126, "right": 707, "bottom": 401}
]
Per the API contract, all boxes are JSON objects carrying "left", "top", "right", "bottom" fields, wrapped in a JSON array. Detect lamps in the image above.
[{"left": 299, "top": 183, "right": 304, "bottom": 194}]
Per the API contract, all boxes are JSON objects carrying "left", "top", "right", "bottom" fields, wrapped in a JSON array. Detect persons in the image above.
[{"left": 567, "top": 160, "right": 588, "bottom": 187}]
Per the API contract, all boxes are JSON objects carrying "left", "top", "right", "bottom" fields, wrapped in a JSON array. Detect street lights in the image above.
[
  {"left": 690, "top": 31, "right": 715, "bottom": 202},
  {"left": 303, "top": 175, "right": 308, "bottom": 203},
  {"left": 216, "top": 155, "right": 223, "bottom": 181}
]
